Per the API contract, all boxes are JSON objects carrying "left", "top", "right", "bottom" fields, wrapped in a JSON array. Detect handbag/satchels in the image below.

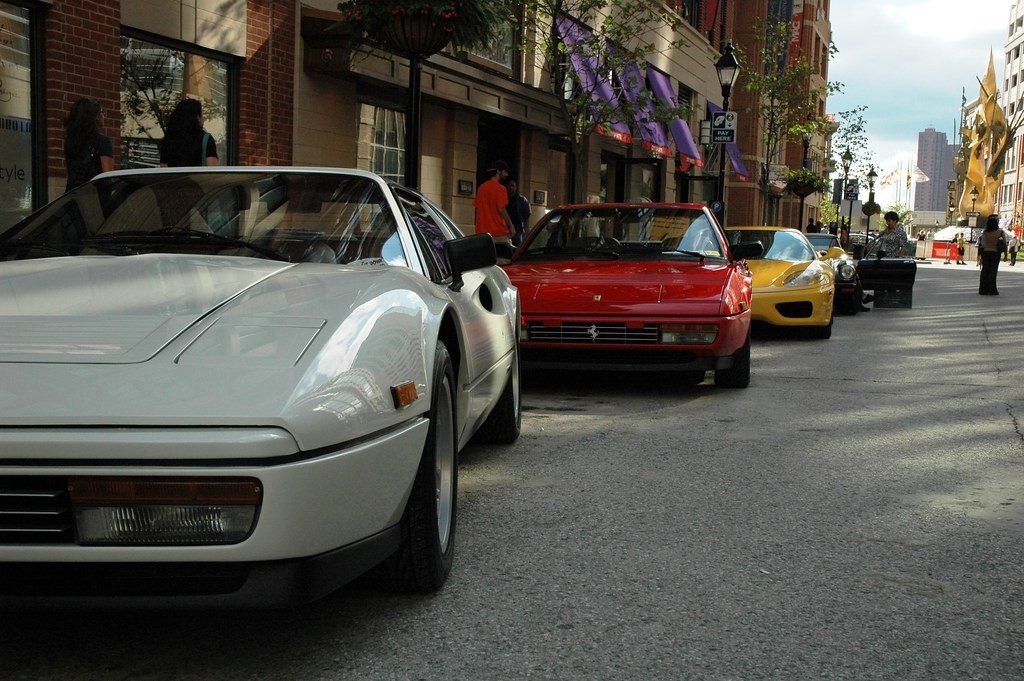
[
  {"left": 1009, "top": 246, "right": 1015, "bottom": 253},
  {"left": 996, "top": 230, "right": 1006, "bottom": 252}
]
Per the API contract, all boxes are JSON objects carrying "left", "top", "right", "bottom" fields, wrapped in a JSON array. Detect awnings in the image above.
[
  {"left": 555, "top": 11, "right": 633, "bottom": 144},
  {"left": 707, "top": 99, "right": 751, "bottom": 182},
  {"left": 607, "top": 40, "right": 674, "bottom": 158},
  {"left": 645, "top": 66, "right": 705, "bottom": 168}
]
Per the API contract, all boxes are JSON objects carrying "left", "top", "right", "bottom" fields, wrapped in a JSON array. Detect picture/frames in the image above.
[
  {"left": 534, "top": 190, "right": 545, "bottom": 204},
  {"left": 458, "top": 179, "right": 474, "bottom": 195}
]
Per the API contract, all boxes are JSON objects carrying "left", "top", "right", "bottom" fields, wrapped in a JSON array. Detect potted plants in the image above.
[
  {"left": 862, "top": 201, "right": 881, "bottom": 217},
  {"left": 779, "top": 166, "right": 834, "bottom": 197}
]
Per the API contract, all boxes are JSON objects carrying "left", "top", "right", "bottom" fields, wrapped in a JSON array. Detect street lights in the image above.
[
  {"left": 840, "top": 144, "right": 853, "bottom": 239},
  {"left": 799, "top": 112, "right": 816, "bottom": 234},
  {"left": 969, "top": 185, "right": 979, "bottom": 242},
  {"left": 936, "top": 201, "right": 956, "bottom": 232},
  {"left": 714, "top": 43, "right": 740, "bottom": 223},
  {"left": 864, "top": 163, "right": 879, "bottom": 244}
]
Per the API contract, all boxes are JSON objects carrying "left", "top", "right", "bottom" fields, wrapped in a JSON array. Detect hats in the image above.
[{"left": 485, "top": 160, "right": 509, "bottom": 172}]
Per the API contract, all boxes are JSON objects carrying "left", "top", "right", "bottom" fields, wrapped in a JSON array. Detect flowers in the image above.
[{"left": 322, "top": 0, "right": 514, "bottom": 71}]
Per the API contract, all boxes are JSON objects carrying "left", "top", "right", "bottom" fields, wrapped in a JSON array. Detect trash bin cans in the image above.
[
  {"left": 853, "top": 243, "right": 864, "bottom": 259},
  {"left": 494, "top": 242, "right": 518, "bottom": 265}
]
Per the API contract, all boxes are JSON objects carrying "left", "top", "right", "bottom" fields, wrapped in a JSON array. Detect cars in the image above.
[
  {"left": 802, "top": 232, "right": 916, "bottom": 316},
  {"left": 847, "top": 235, "right": 875, "bottom": 252},
  {"left": 0, "top": 167, "right": 523, "bottom": 609},
  {"left": 724, "top": 225, "right": 845, "bottom": 339},
  {"left": 494, "top": 202, "right": 764, "bottom": 388}
]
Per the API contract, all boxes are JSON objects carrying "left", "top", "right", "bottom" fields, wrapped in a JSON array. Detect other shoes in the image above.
[
  {"left": 863, "top": 293, "right": 874, "bottom": 304},
  {"left": 859, "top": 305, "right": 871, "bottom": 312}
]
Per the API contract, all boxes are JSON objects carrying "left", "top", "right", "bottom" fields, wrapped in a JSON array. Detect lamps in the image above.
[{"left": 698, "top": 119, "right": 711, "bottom": 146}]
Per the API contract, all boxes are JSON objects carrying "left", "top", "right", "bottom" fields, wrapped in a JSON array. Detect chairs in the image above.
[
  {"left": 567, "top": 237, "right": 620, "bottom": 250},
  {"left": 660, "top": 237, "right": 715, "bottom": 250}
]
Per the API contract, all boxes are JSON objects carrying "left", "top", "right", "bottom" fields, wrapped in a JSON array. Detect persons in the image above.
[
  {"left": 975, "top": 214, "right": 1008, "bottom": 295},
  {"left": 859, "top": 290, "right": 875, "bottom": 312},
  {"left": 1009, "top": 235, "right": 1021, "bottom": 266},
  {"left": 471, "top": 159, "right": 516, "bottom": 266},
  {"left": 159, "top": 98, "right": 219, "bottom": 167},
  {"left": 957, "top": 233, "right": 969, "bottom": 264},
  {"left": 948, "top": 234, "right": 959, "bottom": 264},
  {"left": 505, "top": 175, "right": 532, "bottom": 247},
  {"left": 62, "top": 97, "right": 114, "bottom": 197},
  {"left": 917, "top": 228, "right": 935, "bottom": 240},
  {"left": 878, "top": 211, "right": 907, "bottom": 258},
  {"left": 805, "top": 218, "right": 828, "bottom": 234}
]
